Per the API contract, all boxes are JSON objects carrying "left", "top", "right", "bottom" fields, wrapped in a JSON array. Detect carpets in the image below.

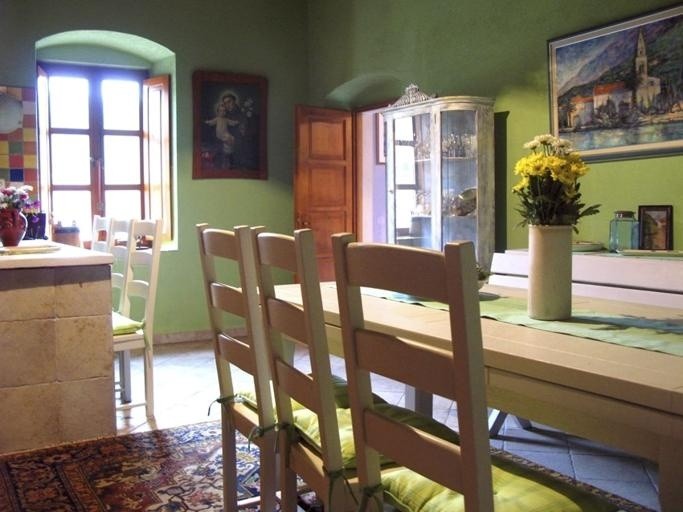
[{"left": 0, "top": 418, "right": 657, "bottom": 512}]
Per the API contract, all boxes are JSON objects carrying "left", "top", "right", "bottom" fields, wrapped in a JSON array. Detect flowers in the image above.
[
  {"left": 0, "top": 184, "right": 41, "bottom": 223},
  {"left": 511, "top": 135, "right": 602, "bottom": 234}
]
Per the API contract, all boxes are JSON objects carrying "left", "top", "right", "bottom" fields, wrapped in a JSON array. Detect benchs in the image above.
[{"left": 487, "top": 253, "right": 683, "bottom": 438}]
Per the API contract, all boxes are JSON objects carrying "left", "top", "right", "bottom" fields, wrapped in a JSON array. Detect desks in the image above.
[
  {"left": 0, "top": 240, "right": 117, "bottom": 454},
  {"left": 229, "top": 281, "right": 683, "bottom": 512}
]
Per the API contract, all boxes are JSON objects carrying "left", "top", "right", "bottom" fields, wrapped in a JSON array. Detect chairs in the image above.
[{"left": 91, "top": 215, "right": 163, "bottom": 417}]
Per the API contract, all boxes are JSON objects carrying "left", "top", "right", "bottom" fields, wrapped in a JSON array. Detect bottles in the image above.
[
  {"left": 70, "top": 217, "right": 77, "bottom": 229},
  {"left": 607, "top": 209, "right": 639, "bottom": 250},
  {"left": 56, "top": 219, "right": 62, "bottom": 228}
]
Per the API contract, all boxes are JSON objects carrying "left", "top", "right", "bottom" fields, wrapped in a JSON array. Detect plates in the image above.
[
  {"left": 570, "top": 241, "right": 601, "bottom": 253},
  {"left": 0, "top": 245, "right": 59, "bottom": 255},
  {"left": 616, "top": 246, "right": 682, "bottom": 258}
]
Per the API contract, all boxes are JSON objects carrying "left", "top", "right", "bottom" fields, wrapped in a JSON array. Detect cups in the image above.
[{"left": 83, "top": 240, "right": 91, "bottom": 250}]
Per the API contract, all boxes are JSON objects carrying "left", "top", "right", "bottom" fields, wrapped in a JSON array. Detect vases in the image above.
[
  {"left": 527, "top": 224, "right": 573, "bottom": 320},
  {"left": 1, "top": 208, "right": 28, "bottom": 246}
]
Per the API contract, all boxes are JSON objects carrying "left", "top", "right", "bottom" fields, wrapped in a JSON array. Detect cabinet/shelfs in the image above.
[{"left": 381, "top": 95, "right": 495, "bottom": 275}]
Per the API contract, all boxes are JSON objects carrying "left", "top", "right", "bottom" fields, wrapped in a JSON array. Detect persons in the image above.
[{"left": 200, "top": 89, "right": 250, "bottom": 168}]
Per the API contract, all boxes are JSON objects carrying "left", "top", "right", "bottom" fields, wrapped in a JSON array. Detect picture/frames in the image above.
[
  {"left": 192, "top": 71, "right": 269, "bottom": 181},
  {"left": 375, "top": 113, "right": 386, "bottom": 165},
  {"left": 639, "top": 205, "right": 673, "bottom": 250},
  {"left": 547, "top": 3, "right": 683, "bottom": 161}
]
